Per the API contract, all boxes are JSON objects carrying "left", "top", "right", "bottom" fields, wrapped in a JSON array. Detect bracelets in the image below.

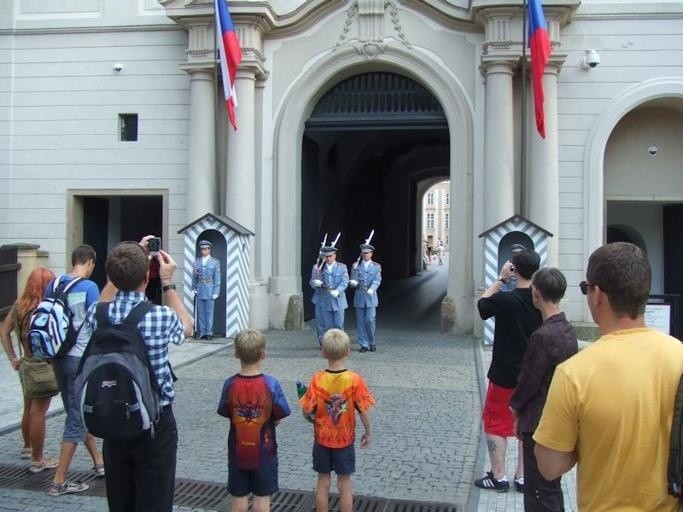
[
  {"left": 163, "top": 284, "right": 176, "bottom": 293},
  {"left": 9, "top": 354, "right": 16, "bottom": 361}
]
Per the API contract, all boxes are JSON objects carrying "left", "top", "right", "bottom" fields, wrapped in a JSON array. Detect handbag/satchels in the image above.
[{"left": 19, "top": 356, "right": 60, "bottom": 399}]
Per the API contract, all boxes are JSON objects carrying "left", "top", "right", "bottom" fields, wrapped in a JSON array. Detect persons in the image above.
[
  {"left": 192, "top": 240, "right": 221, "bottom": 340},
  {"left": 216, "top": 328, "right": 292, "bottom": 512},
  {"left": 43, "top": 244, "right": 105, "bottom": 497},
  {"left": 532, "top": 241, "right": 682, "bottom": 512},
  {"left": 425, "top": 237, "right": 445, "bottom": 266},
  {"left": 298, "top": 328, "right": 376, "bottom": 512},
  {"left": 475, "top": 249, "right": 543, "bottom": 493},
  {"left": 508, "top": 266, "right": 578, "bottom": 512},
  {"left": 85, "top": 234, "right": 194, "bottom": 512},
  {"left": 309, "top": 244, "right": 382, "bottom": 353},
  {"left": 0, "top": 267, "right": 60, "bottom": 474}
]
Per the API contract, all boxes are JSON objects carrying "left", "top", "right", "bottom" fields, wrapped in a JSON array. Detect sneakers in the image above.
[
  {"left": 513, "top": 473, "right": 524, "bottom": 493},
  {"left": 474, "top": 470, "right": 509, "bottom": 493}
]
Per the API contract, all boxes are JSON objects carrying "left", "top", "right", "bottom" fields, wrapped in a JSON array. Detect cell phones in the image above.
[{"left": 148, "top": 237, "right": 162, "bottom": 257}]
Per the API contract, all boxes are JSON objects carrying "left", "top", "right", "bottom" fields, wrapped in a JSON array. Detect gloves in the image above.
[
  {"left": 212, "top": 293, "right": 219, "bottom": 299},
  {"left": 192, "top": 290, "right": 196, "bottom": 297},
  {"left": 312, "top": 279, "right": 373, "bottom": 297}
]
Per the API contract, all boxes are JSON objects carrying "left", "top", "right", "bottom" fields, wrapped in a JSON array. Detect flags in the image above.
[
  {"left": 215, "top": 0, "right": 243, "bottom": 131},
  {"left": 526, "top": 0, "right": 552, "bottom": 139}
]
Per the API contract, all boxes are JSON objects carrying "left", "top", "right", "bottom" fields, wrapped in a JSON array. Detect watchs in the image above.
[{"left": 497, "top": 276, "right": 505, "bottom": 284}]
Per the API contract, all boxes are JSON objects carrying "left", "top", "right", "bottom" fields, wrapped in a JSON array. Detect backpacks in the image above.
[
  {"left": 74, "top": 300, "right": 163, "bottom": 442},
  {"left": 26, "top": 275, "right": 88, "bottom": 360}
]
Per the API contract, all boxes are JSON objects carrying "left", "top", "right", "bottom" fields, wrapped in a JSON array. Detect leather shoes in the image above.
[
  {"left": 195, "top": 334, "right": 213, "bottom": 339},
  {"left": 359, "top": 345, "right": 377, "bottom": 352}
]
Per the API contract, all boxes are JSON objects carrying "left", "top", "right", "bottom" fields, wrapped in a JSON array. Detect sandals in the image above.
[{"left": 21, "top": 447, "right": 106, "bottom": 497}]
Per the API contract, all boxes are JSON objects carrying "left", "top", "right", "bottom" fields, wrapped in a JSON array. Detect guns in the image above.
[
  {"left": 315, "top": 233, "right": 340, "bottom": 282},
  {"left": 193, "top": 296, "right": 197, "bottom": 340},
  {"left": 353, "top": 230, "right": 374, "bottom": 270},
  {"left": 314, "top": 233, "right": 328, "bottom": 281}
]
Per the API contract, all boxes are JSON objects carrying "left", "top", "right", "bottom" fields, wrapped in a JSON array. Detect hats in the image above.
[
  {"left": 199, "top": 240, "right": 215, "bottom": 249},
  {"left": 320, "top": 244, "right": 375, "bottom": 255},
  {"left": 512, "top": 244, "right": 525, "bottom": 253}
]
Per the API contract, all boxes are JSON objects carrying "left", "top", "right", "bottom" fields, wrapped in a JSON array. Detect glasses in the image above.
[{"left": 579, "top": 281, "right": 594, "bottom": 294}]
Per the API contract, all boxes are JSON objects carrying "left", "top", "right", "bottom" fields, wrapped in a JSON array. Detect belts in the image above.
[{"left": 198, "top": 280, "right": 213, "bottom": 283}]
